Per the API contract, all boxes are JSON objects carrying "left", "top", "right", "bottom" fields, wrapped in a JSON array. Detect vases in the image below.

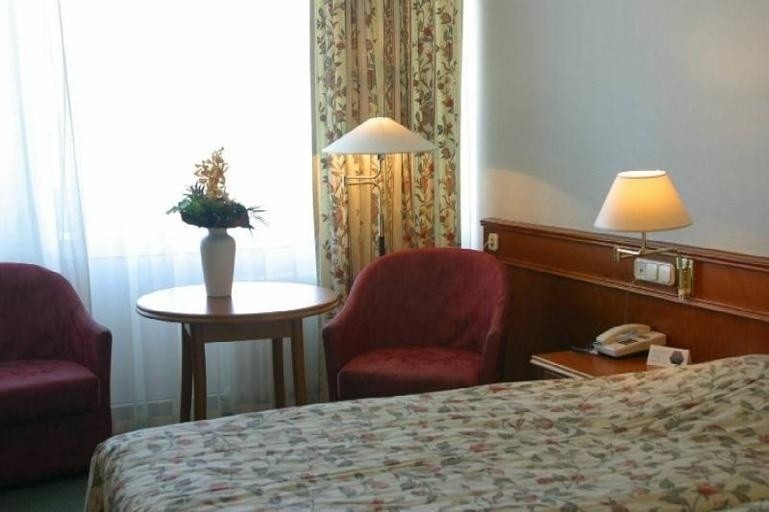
[{"left": 201, "top": 227, "right": 236, "bottom": 298}]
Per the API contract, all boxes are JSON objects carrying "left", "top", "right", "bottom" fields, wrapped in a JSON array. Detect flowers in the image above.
[{"left": 165, "top": 146, "right": 266, "bottom": 230}]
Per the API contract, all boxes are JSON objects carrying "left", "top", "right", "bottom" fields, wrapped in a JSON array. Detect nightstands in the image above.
[{"left": 529, "top": 351, "right": 661, "bottom": 378}]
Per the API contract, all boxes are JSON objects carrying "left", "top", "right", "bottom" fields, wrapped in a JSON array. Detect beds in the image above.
[{"left": 84, "top": 353, "right": 769, "bottom": 512}]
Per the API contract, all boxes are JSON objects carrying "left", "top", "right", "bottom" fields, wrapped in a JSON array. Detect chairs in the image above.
[
  {"left": 1, "top": 263, "right": 112, "bottom": 484},
  {"left": 322, "top": 248, "right": 509, "bottom": 401}
]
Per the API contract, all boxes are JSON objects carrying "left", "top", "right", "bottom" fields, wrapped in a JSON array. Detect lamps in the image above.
[
  {"left": 592, "top": 171, "right": 693, "bottom": 260},
  {"left": 321, "top": 116, "right": 439, "bottom": 257}
]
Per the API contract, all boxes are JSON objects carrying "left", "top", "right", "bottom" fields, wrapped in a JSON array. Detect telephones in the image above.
[{"left": 592, "top": 323, "right": 667, "bottom": 358}]
